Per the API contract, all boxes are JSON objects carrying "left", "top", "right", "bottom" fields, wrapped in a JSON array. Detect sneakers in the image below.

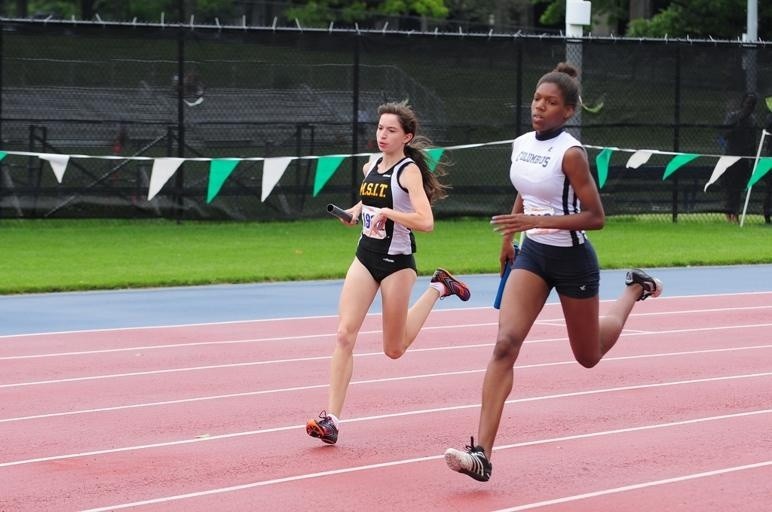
[
  {"left": 430, "top": 267, "right": 470, "bottom": 302},
  {"left": 306, "top": 417, "right": 338, "bottom": 444},
  {"left": 443, "top": 444, "right": 492, "bottom": 481},
  {"left": 624, "top": 268, "right": 663, "bottom": 301}
]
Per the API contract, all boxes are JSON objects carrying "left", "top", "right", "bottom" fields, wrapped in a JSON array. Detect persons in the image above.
[
  {"left": 305, "top": 101, "right": 473, "bottom": 446},
  {"left": 763, "top": 112, "right": 772, "bottom": 224},
  {"left": 718, "top": 91, "right": 758, "bottom": 223},
  {"left": 443, "top": 62, "right": 665, "bottom": 482}
]
